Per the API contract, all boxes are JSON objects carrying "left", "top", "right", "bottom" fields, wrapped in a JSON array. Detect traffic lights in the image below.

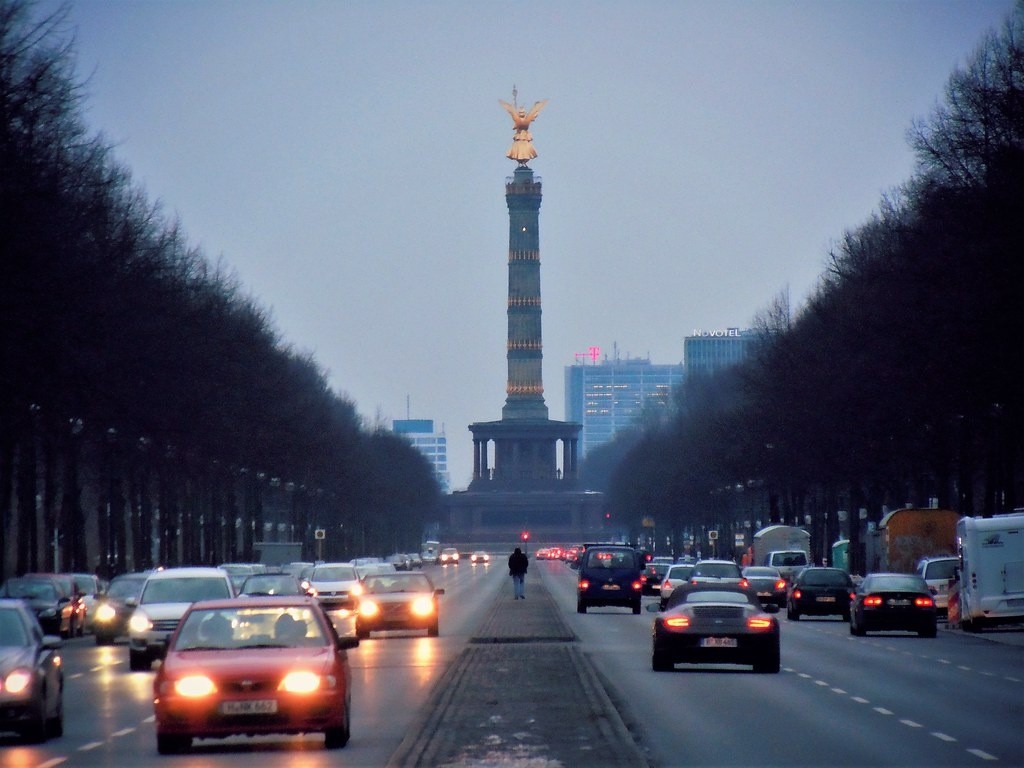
[{"left": 523, "top": 534, "right": 529, "bottom": 539}]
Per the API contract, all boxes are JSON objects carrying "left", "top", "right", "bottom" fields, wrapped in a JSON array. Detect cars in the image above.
[
  {"left": 645, "top": 549, "right": 809, "bottom": 613},
  {"left": 787, "top": 567, "right": 857, "bottom": 622},
  {"left": 218, "top": 542, "right": 460, "bottom": 605},
  {"left": 850, "top": 572, "right": 938, "bottom": 640},
  {"left": 535, "top": 547, "right": 576, "bottom": 562},
  {"left": 152, "top": 596, "right": 360, "bottom": 755},
  {"left": 355, "top": 573, "right": 445, "bottom": 638},
  {"left": 0, "top": 599, "right": 66, "bottom": 744},
  {"left": 646, "top": 581, "right": 781, "bottom": 673},
  {"left": 1, "top": 572, "right": 158, "bottom": 646},
  {"left": 470, "top": 550, "right": 490, "bottom": 563}
]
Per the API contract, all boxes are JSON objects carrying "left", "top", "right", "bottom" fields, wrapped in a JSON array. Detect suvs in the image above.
[
  {"left": 126, "top": 567, "right": 236, "bottom": 671},
  {"left": 916, "top": 556, "right": 960, "bottom": 613}
]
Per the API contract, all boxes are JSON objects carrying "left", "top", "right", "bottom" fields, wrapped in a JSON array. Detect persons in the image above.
[
  {"left": 508, "top": 548, "right": 529, "bottom": 600},
  {"left": 498, "top": 100, "right": 551, "bottom": 167}
]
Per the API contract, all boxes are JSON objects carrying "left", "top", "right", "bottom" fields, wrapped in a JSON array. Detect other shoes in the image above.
[
  {"left": 521, "top": 595, "right": 526, "bottom": 598},
  {"left": 514, "top": 597, "right": 519, "bottom": 600}
]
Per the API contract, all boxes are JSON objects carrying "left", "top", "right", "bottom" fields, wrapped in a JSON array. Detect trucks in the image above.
[{"left": 957, "top": 514, "right": 1024, "bottom": 629}]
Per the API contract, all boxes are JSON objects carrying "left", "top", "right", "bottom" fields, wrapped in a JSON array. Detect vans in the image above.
[{"left": 575, "top": 544, "right": 642, "bottom": 617}]
